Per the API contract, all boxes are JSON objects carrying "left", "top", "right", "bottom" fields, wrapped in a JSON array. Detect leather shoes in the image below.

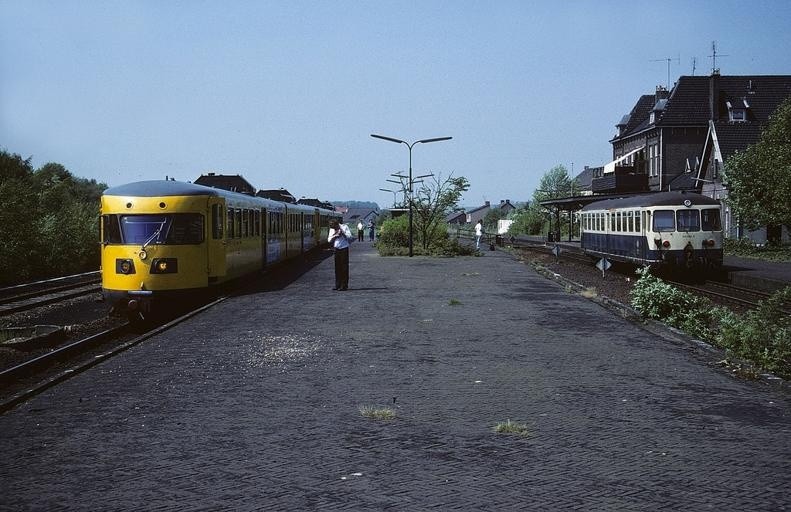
[{"left": 331, "top": 287, "right": 347, "bottom": 291}]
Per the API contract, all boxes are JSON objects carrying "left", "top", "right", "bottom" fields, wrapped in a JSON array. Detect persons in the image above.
[
  {"left": 474, "top": 218, "right": 484, "bottom": 251},
  {"left": 356, "top": 218, "right": 366, "bottom": 242},
  {"left": 326, "top": 215, "right": 353, "bottom": 291},
  {"left": 367, "top": 218, "right": 376, "bottom": 242}
]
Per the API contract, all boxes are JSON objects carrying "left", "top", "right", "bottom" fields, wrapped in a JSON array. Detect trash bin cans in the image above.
[
  {"left": 548, "top": 231, "right": 557, "bottom": 242},
  {"left": 496, "top": 235, "right": 501, "bottom": 244}
]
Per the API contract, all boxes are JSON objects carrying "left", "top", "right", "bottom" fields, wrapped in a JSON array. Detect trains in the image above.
[
  {"left": 577, "top": 189, "right": 724, "bottom": 276},
  {"left": 98, "top": 173, "right": 344, "bottom": 322}
]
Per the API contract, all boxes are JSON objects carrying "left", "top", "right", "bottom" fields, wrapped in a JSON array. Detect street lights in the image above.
[{"left": 369, "top": 134, "right": 453, "bottom": 258}]
